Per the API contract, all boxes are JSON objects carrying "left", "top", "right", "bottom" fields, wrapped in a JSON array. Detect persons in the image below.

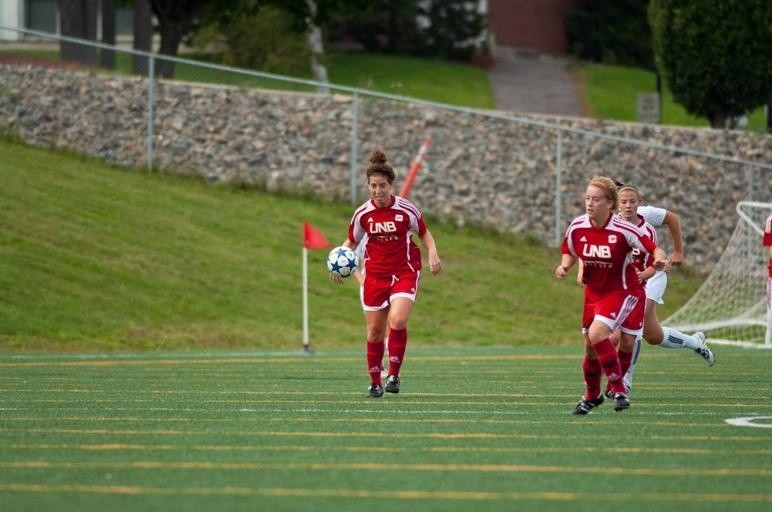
[
  {"left": 577, "top": 182, "right": 658, "bottom": 398},
  {"left": 557, "top": 175, "right": 668, "bottom": 414},
  {"left": 351, "top": 229, "right": 403, "bottom": 381},
  {"left": 610, "top": 178, "right": 717, "bottom": 393},
  {"left": 763, "top": 213, "right": 772, "bottom": 311},
  {"left": 329, "top": 150, "right": 442, "bottom": 398}
]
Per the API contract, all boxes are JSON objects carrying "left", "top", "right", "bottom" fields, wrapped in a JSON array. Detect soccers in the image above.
[{"left": 328, "top": 246, "right": 358, "bottom": 276}]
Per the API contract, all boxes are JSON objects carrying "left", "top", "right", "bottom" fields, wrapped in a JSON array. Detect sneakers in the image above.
[
  {"left": 368, "top": 374, "right": 399, "bottom": 397},
  {"left": 693, "top": 332, "right": 714, "bottom": 367},
  {"left": 572, "top": 387, "right": 630, "bottom": 415}
]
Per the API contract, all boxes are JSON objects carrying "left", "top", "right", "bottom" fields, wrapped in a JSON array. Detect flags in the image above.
[{"left": 305, "top": 221, "right": 335, "bottom": 251}]
